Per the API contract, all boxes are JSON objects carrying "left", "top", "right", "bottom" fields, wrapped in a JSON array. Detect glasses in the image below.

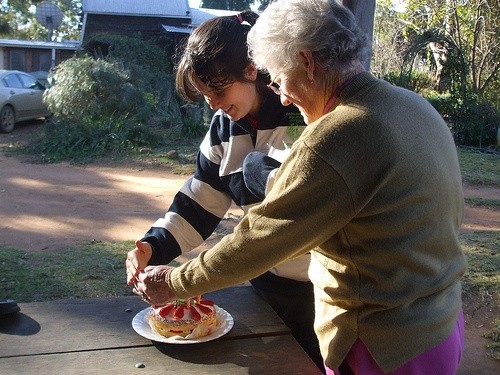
[{"left": 266, "top": 63, "right": 288, "bottom": 95}]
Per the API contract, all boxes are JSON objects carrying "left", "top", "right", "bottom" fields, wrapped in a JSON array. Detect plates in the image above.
[{"left": 131, "top": 304, "right": 234, "bottom": 344}]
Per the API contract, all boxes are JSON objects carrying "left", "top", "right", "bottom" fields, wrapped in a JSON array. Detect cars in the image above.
[{"left": 0, "top": 70, "right": 51, "bottom": 134}]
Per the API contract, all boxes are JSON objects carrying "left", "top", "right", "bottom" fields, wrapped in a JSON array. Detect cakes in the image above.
[{"left": 148, "top": 295, "right": 217, "bottom": 337}]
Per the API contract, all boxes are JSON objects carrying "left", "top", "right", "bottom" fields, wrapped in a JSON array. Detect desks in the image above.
[{"left": 0, "top": 286, "right": 325, "bottom": 375}]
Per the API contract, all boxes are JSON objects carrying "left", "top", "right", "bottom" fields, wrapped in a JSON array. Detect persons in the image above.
[
  {"left": 126, "top": 10, "right": 327, "bottom": 375},
  {"left": 133, "top": 0, "right": 467, "bottom": 375}
]
